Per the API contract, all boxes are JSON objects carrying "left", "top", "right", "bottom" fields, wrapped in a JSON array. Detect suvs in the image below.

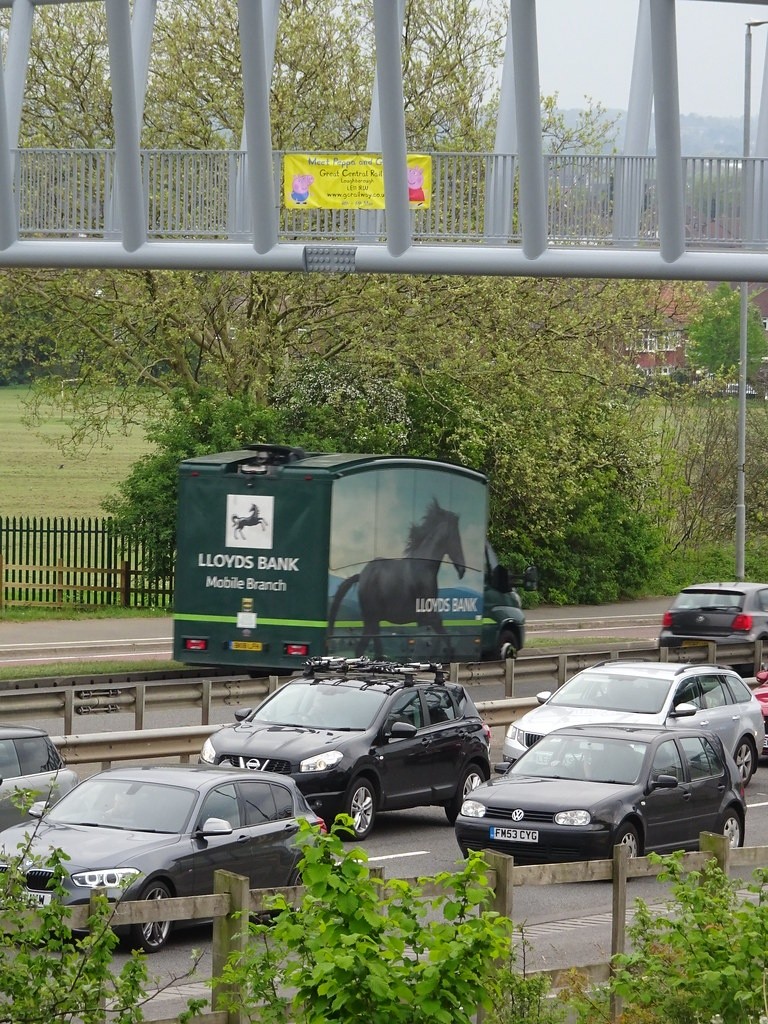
[
  {"left": 199, "top": 654, "right": 493, "bottom": 841},
  {"left": 503, "top": 657, "right": 766, "bottom": 790}
]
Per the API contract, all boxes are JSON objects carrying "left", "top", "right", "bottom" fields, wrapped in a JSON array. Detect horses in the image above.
[
  {"left": 327, "top": 496, "right": 466, "bottom": 661},
  {"left": 232, "top": 504, "right": 268, "bottom": 540}
]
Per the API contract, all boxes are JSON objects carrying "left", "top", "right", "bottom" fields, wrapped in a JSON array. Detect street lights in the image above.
[{"left": 733, "top": 15, "right": 768, "bottom": 581}]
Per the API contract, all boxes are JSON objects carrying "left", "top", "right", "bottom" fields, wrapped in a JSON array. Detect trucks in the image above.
[{"left": 170, "top": 443, "right": 526, "bottom": 683}]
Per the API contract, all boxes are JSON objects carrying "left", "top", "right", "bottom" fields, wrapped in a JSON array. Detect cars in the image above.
[
  {"left": 0, "top": 720, "right": 82, "bottom": 839},
  {"left": 0, "top": 763, "right": 330, "bottom": 955},
  {"left": 659, "top": 580, "right": 768, "bottom": 666},
  {"left": 722, "top": 382, "right": 756, "bottom": 396},
  {"left": 456, "top": 721, "right": 749, "bottom": 883},
  {"left": 746, "top": 668, "right": 768, "bottom": 759}
]
